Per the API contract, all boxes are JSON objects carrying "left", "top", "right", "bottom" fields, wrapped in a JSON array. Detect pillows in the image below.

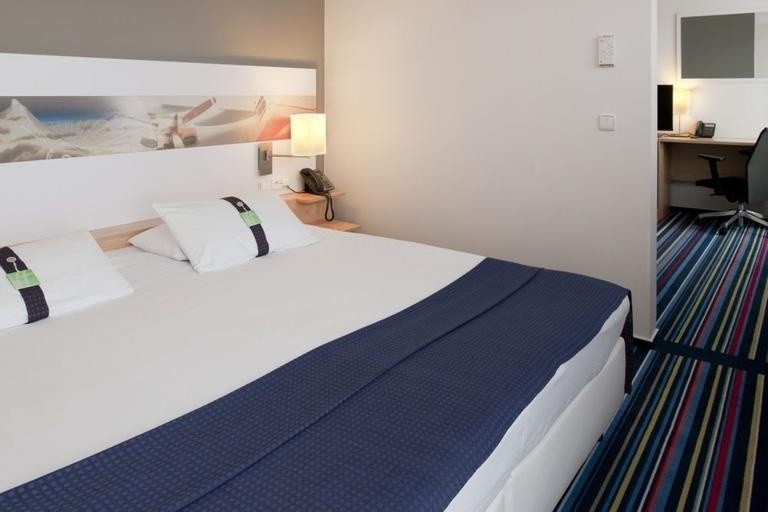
[
  {"left": 152, "top": 189, "right": 322, "bottom": 275},
  {"left": 0, "top": 227, "right": 137, "bottom": 330},
  {"left": 127, "top": 223, "right": 190, "bottom": 262}
]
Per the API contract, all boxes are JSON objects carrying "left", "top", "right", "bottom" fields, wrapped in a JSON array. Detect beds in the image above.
[{"left": 0, "top": 223, "right": 634, "bottom": 512}]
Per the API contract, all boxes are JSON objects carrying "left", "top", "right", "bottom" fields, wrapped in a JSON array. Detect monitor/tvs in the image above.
[{"left": 657, "top": 84, "right": 674, "bottom": 132}]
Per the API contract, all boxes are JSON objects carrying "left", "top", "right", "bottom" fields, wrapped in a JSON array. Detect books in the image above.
[{"left": 661, "top": 134, "right": 690, "bottom": 140}]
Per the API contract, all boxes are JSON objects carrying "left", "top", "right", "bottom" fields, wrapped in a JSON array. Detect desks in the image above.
[{"left": 658, "top": 133, "right": 758, "bottom": 225}]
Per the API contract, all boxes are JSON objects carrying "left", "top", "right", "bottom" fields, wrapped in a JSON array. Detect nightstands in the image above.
[{"left": 279, "top": 190, "right": 361, "bottom": 233}]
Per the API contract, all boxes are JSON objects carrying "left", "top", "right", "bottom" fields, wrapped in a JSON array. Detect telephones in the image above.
[
  {"left": 300, "top": 168, "right": 335, "bottom": 196},
  {"left": 694, "top": 121, "right": 716, "bottom": 138}
]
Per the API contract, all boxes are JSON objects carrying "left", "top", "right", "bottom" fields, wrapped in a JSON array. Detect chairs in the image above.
[{"left": 695, "top": 127, "right": 768, "bottom": 236}]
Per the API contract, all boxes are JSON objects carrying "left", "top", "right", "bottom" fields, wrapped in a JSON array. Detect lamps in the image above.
[{"left": 257, "top": 112, "right": 327, "bottom": 176}]
